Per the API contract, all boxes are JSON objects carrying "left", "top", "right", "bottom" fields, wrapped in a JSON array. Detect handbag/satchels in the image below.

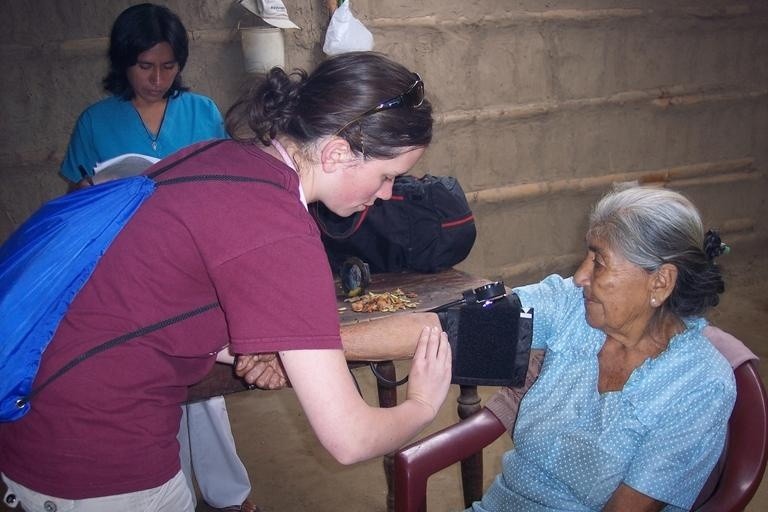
[{"left": 308, "top": 175, "right": 477, "bottom": 273}]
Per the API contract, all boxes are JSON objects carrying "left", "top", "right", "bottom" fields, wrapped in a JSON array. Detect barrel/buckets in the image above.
[{"left": 237, "top": 12, "right": 286, "bottom": 75}]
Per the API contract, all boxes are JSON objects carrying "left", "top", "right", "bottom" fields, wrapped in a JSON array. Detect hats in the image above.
[{"left": 240, "top": 0, "right": 299, "bottom": 29}]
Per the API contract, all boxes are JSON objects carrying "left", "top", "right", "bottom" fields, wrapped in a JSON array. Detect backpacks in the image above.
[{"left": 1, "top": 174, "right": 161, "bottom": 423}]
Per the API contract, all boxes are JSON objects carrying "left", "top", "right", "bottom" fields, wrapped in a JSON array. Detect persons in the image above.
[
  {"left": 235, "top": 185, "right": 737, "bottom": 510},
  {"left": 1, "top": 53, "right": 454, "bottom": 512},
  {"left": 56, "top": 3, "right": 256, "bottom": 512}
]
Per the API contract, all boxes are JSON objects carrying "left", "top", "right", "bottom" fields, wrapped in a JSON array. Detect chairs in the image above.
[{"left": 382, "top": 319, "right": 767, "bottom": 512}]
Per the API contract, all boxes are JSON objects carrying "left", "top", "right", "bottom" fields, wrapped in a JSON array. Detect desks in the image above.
[{"left": 179, "top": 268, "right": 492, "bottom": 507}]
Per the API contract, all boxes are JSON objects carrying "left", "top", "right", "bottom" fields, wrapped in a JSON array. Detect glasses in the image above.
[{"left": 330, "top": 71, "right": 425, "bottom": 137}]
